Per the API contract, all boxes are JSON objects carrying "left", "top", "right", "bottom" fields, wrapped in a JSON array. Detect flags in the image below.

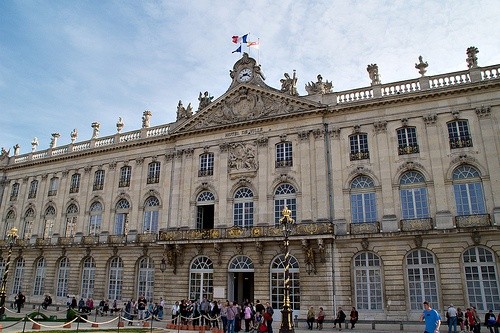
[
  {"left": 232, "top": 46, "right": 241, "bottom": 53},
  {"left": 247, "top": 40, "right": 259, "bottom": 49},
  {"left": 232, "top": 34, "right": 248, "bottom": 43}
]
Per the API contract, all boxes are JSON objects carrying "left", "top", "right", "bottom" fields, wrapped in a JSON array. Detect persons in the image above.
[
  {"left": 317, "top": 307, "right": 324, "bottom": 330},
  {"left": 14, "top": 291, "right": 26, "bottom": 313},
  {"left": 123, "top": 295, "right": 165, "bottom": 325},
  {"left": 420, "top": 301, "right": 441, "bottom": 333},
  {"left": 312, "top": 74, "right": 332, "bottom": 93},
  {"left": 350, "top": 307, "right": 358, "bottom": 329},
  {"left": 306, "top": 306, "right": 316, "bottom": 330},
  {"left": 66, "top": 296, "right": 93, "bottom": 313},
  {"left": 177, "top": 103, "right": 187, "bottom": 119},
  {"left": 446, "top": 304, "right": 500, "bottom": 333},
  {"left": 99, "top": 298, "right": 117, "bottom": 317},
  {"left": 42, "top": 296, "right": 52, "bottom": 309},
  {"left": 198, "top": 91, "right": 214, "bottom": 107},
  {"left": 280, "top": 73, "right": 297, "bottom": 92},
  {"left": 333, "top": 307, "right": 346, "bottom": 331},
  {"left": 171, "top": 297, "right": 273, "bottom": 333}
]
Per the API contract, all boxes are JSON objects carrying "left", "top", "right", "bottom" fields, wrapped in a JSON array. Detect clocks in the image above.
[{"left": 239, "top": 68, "right": 252, "bottom": 82}]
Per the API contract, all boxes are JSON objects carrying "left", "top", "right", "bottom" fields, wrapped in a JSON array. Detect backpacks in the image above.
[{"left": 258, "top": 324, "right": 267, "bottom": 332}]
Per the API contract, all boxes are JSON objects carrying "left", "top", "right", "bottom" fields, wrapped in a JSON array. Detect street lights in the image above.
[
  {"left": 0, "top": 227, "right": 20, "bottom": 319},
  {"left": 279, "top": 206, "right": 298, "bottom": 333}
]
